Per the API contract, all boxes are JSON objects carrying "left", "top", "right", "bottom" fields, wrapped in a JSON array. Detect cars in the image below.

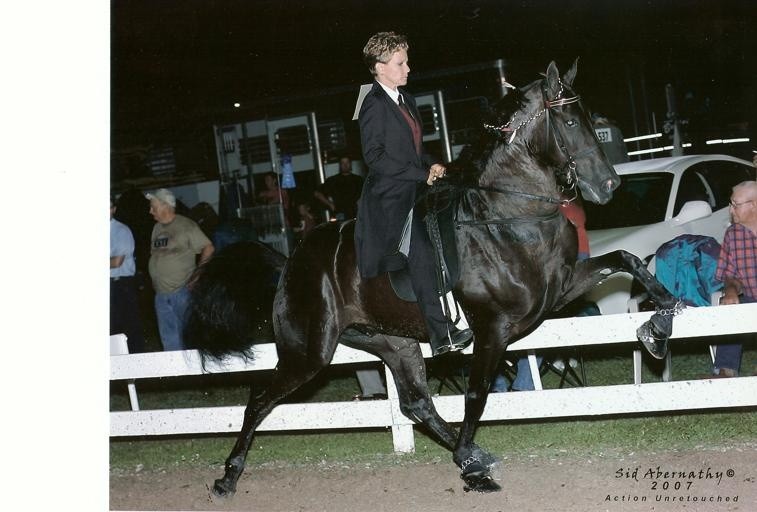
[{"left": 551, "top": 152, "right": 756, "bottom": 317}]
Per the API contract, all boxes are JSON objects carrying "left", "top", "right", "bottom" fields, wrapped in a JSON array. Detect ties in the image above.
[{"left": 398, "top": 94, "right": 418, "bottom": 127}]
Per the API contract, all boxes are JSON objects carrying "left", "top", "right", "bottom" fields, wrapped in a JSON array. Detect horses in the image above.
[{"left": 179, "top": 56, "right": 679, "bottom": 500}]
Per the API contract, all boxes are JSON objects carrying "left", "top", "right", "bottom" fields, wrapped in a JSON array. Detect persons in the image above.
[
  {"left": 110, "top": 196, "right": 140, "bottom": 348},
  {"left": 351, "top": 363, "right": 389, "bottom": 399},
  {"left": 483, "top": 348, "right": 554, "bottom": 395},
  {"left": 257, "top": 170, "right": 292, "bottom": 228},
  {"left": 291, "top": 200, "right": 318, "bottom": 235},
  {"left": 592, "top": 112, "right": 630, "bottom": 167},
  {"left": 144, "top": 187, "right": 216, "bottom": 352},
  {"left": 312, "top": 153, "right": 364, "bottom": 222},
  {"left": 697, "top": 179, "right": 757, "bottom": 378},
  {"left": 550, "top": 190, "right": 592, "bottom": 371},
  {"left": 348, "top": 30, "right": 473, "bottom": 355}
]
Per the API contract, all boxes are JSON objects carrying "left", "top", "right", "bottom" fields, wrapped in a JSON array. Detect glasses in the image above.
[{"left": 728, "top": 200, "right": 755, "bottom": 207}]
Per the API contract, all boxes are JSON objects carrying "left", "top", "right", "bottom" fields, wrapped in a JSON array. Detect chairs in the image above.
[{"left": 627, "top": 254, "right": 724, "bottom": 384}]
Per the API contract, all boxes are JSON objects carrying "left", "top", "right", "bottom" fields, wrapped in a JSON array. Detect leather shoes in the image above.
[{"left": 435, "top": 326, "right": 473, "bottom": 354}]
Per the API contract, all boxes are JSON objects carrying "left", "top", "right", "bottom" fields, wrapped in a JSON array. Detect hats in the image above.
[{"left": 146, "top": 189, "right": 175, "bottom": 208}]
